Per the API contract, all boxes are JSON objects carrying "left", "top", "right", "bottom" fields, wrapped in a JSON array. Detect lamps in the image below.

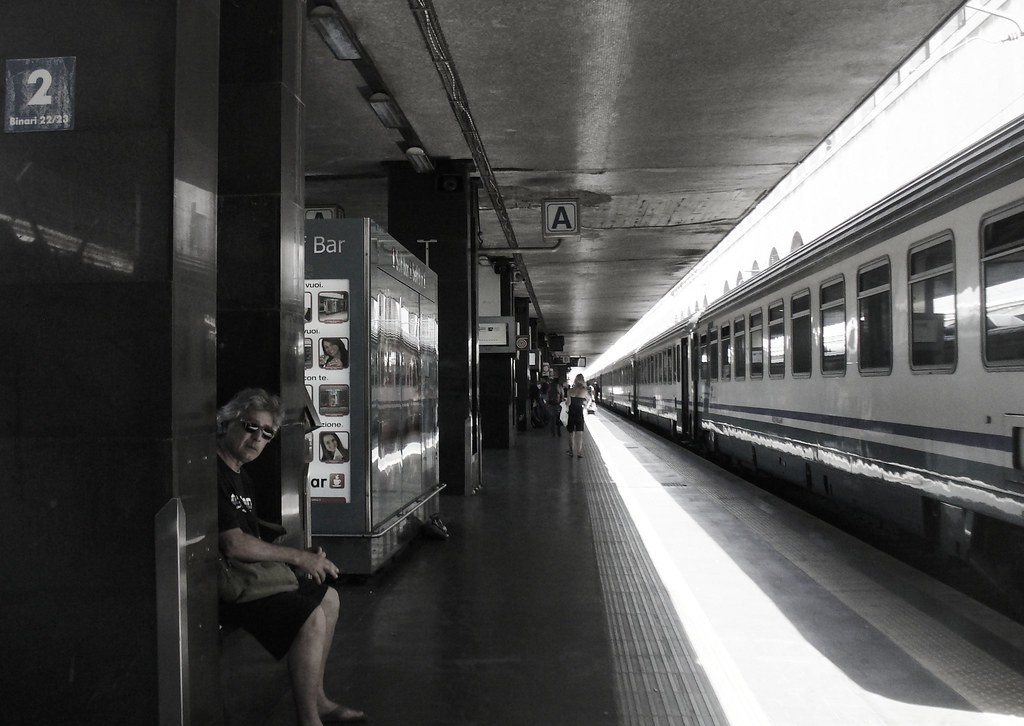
[
  {"left": 368, "top": 91, "right": 408, "bottom": 129},
  {"left": 308, "top": 5, "right": 363, "bottom": 62},
  {"left": 405, "top": 147, "right": 435, "bottom": 173}
]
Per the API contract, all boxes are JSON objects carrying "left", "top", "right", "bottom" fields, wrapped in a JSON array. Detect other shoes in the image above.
[
  {"left": 323, "top": 703, "right": 365, "bottom": 725},
  {"left": 566, "top": 449, "right": 573, "bottom": 456},
  {"left": 576, "top": 451, "right": 585, "bottom": 458}
]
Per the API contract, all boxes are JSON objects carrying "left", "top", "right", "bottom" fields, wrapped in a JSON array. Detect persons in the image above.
[
  {"left": 320, "top": 337, "right": 349, "bottom": 367},
  {"left": 320, "top": 434, "right": 349, "bottom": 461},
  {"left": 565, "top": 374, "right": 592, "bottom": 458},
  {"left": 216, "top": 388, "right": 363, "bottom": 726},
  {"left": 586, "top": 382, "right": 599, "bottom": 405},
  {"left": 531, "top": 375, "right": 564, "bottom": 437}
]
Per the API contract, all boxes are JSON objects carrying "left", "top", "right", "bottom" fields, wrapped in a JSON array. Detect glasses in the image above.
[{"left": 240, "top": 416, "right": 275, "bottom": 442}]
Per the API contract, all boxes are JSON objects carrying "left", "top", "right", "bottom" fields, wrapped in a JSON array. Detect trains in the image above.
[{"left": 583, "top": 113, "right": 1024, "bottom": 587}]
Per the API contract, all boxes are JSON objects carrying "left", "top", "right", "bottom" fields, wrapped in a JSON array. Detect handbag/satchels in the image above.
[{"left": 217, "top": 545, "right": 301, "bottom": 604}]
